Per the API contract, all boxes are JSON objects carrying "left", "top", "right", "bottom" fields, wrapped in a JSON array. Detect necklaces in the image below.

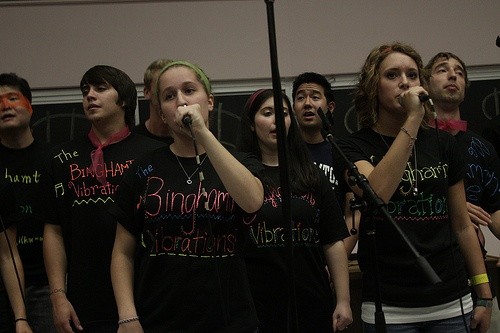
[
  {"left": 374, "top": 125, "right": 418, "bottom": 196},
  {"left": 173, "top": 149, "right": 207, "bottom": 185}
]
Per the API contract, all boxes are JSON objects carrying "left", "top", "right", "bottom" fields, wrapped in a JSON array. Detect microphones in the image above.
[
  {"left": 419, "top": 93, "right": 430, "bottom": 102},
  {"left": 182, "top": 115, "right": 192, "bottom": 125}
]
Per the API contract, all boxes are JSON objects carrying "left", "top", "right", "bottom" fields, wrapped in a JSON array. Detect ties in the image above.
[{"left": 87, "top": 124, "right": 130, "bottom": 187}]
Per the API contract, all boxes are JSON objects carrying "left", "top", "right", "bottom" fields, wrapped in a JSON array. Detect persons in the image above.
[{"left": 0, "top": 43, "right": 500, "bottom": 333}]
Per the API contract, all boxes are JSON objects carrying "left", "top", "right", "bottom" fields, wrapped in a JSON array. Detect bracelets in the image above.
[
  {"left": 467, "top": 273, "right": 489, "bottom": 286},
  {"left": 118, "top": 316, "right": 139, "bottom": 325},
  {"left": 15, "top": 318, "right": 27, "bottom": 323},
  {"left": 400, "top": 127, "right": 417, "bottom": 149},
  {"left": 477, "top": 298, "right": 494, "bottom": 307},
  {"left": 49, "top": 288, "right": 65, "bottom": 296}
]
